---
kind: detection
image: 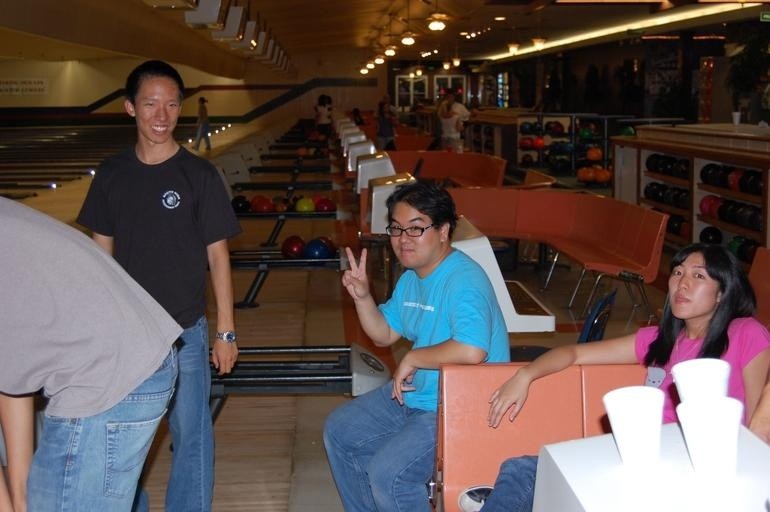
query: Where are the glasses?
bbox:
[386,221,438,237]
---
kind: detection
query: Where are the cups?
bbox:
[732,111,742,127]
[602,385,667,464]
[678,399,744,472]
[670,356,730,398]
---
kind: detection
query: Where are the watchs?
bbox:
[215,329,237,345]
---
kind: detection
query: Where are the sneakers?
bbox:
[457,486,495,512]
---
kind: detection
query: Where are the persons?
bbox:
[191,94,213,152]
[72,58,242,510]
[320,176,512,511]
[311,86,505,155]
[0,190,185,512]
[458,238,770,510]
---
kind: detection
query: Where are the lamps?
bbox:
[355,0,553,83]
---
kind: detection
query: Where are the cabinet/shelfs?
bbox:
[390,75,430,112]
[434,70,466,111]
[612,140,693,281]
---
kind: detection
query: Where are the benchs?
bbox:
[692,142,764,284]
